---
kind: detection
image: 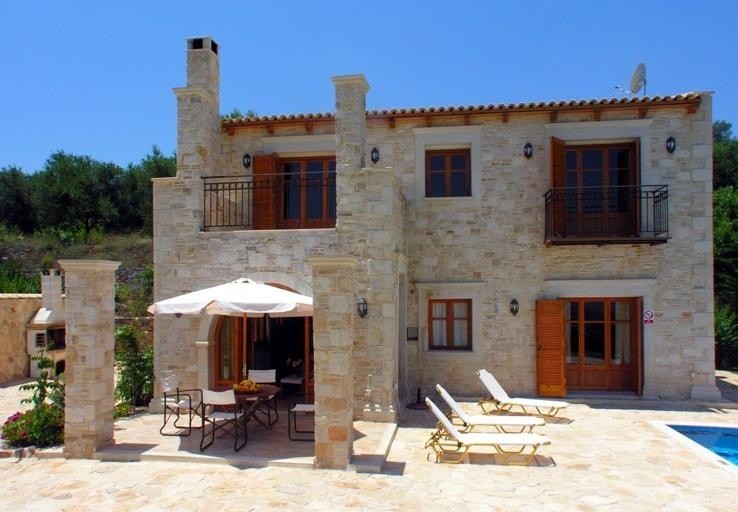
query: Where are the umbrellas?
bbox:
[142,273,313,386]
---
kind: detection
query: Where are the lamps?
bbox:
[357,297,368,318]
[509,299,520,316]
[666,136,675,154]
[243,152,250,168]
[371,146,379,164]
[524,142,533,160]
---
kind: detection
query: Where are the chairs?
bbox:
[159,369,314,452]
[424,367,571,467]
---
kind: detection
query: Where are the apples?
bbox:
[232,384,239,390]
